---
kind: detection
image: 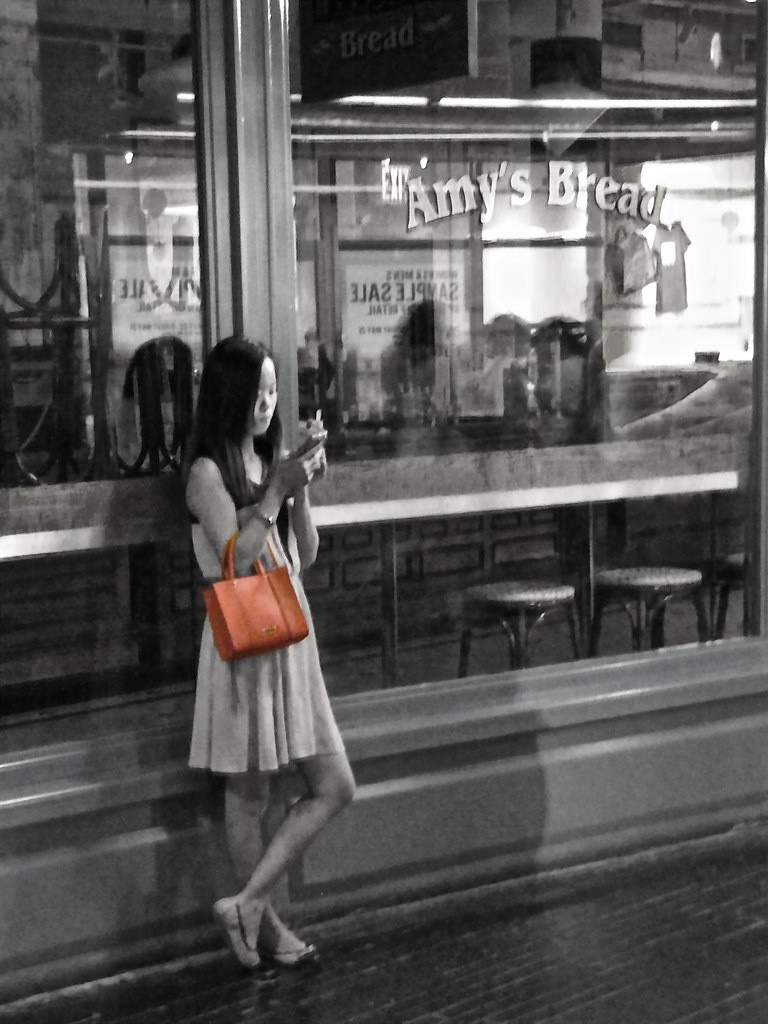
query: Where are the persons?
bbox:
[362,290,634,447]
[172,336,360,979]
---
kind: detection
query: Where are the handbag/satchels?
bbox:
[203,530,309,661]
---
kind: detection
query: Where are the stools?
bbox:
[457,580,584,678]
[714,551,753,640]
[585,565,711,658]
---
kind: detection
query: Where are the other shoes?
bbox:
[213,898,261,969]
[257,941,319,968]
